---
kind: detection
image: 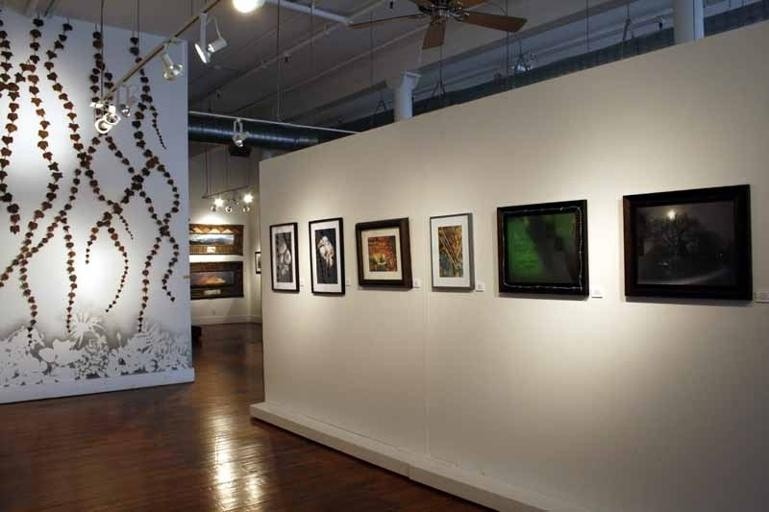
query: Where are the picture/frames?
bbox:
[623,185,752,300]
[355,218,412,288]
[430,213,475,290]
[255,251,261,274]
[270,222,299,292]
[497,200,588,295]
[309,218,345,294]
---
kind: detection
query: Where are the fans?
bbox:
[348,0,527,49]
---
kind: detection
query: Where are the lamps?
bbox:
[202,183,253,214]
[96,0,266,132]
[227,116,250,148]
